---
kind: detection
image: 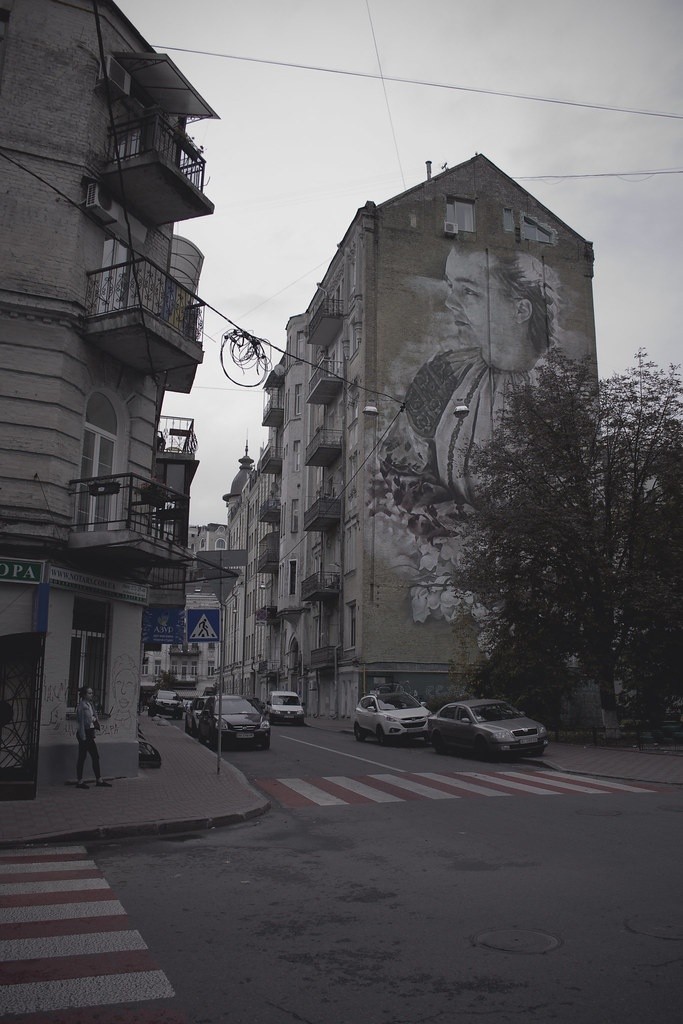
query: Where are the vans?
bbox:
[266,690,306,724]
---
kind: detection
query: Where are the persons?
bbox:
[255,701,270,720]
[381,227,599,624]
[76,685,112,789]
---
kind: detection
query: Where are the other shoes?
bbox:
[96,781,112,787]
[77,782,90,789]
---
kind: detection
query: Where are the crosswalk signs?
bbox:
[188,607,222,642]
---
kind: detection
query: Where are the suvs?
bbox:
[149,689,184,720]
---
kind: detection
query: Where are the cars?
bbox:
[197,695,271,749]
[185,700,192,711]
[184,695,208,737]
[353,691,434,746]
[428,698,548,759]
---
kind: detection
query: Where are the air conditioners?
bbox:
[443,222,460,235]
[85,182,120,223]
[98,53,131,97]
